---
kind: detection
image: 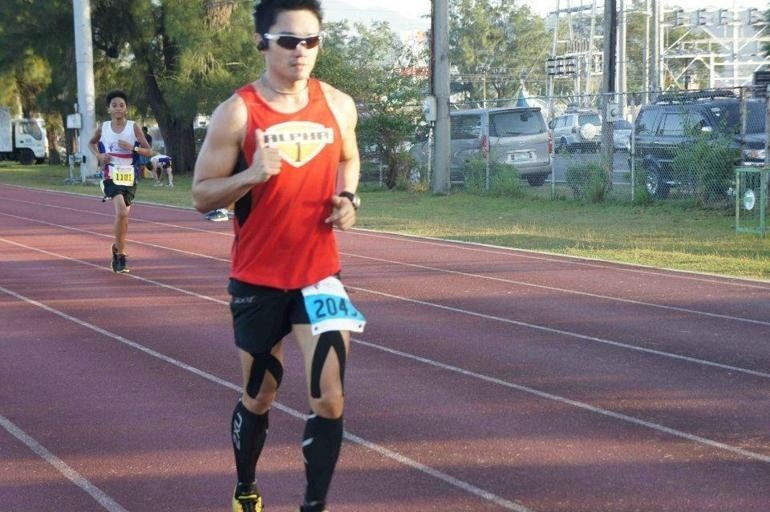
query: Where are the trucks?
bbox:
[0,110,49,166]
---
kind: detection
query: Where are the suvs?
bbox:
[627,86,770,203]
[546,108,609,156]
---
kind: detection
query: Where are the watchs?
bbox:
[338,192,361,210]
[134,146,138,153]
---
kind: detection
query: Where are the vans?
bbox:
[604,117,635,152]
[406,103,555,189]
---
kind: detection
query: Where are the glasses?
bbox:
[263,33,320,49]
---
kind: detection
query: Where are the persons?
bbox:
[88,89,152,274]
[139,127,152,165]
[147,154,175,188]
[191,0,367,512]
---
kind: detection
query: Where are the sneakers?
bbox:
[205,209,229,221]
[231,479,263,512]
[116,252,130,274]
[110,244,117,273]
